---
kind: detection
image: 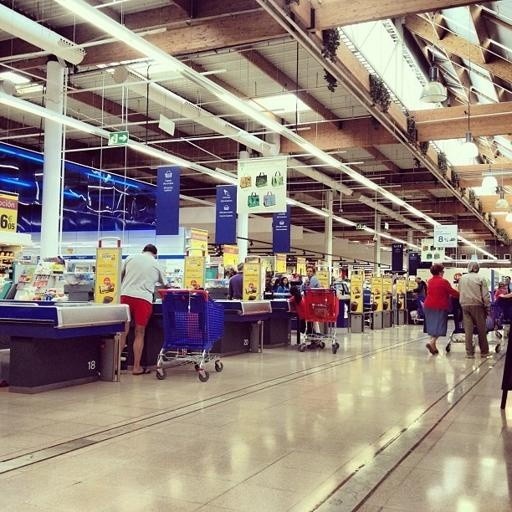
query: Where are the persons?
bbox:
[115,241,170,376]
[451,273,512,340]
[410,278,428,325]
[223,261,322,351]
[422,264,460,355]
[458,261,494,358]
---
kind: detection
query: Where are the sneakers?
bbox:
[426,343,438,355]
[308,343,320,349]
[465,353,491,358]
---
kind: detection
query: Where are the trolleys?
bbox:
[154,289,228,382]
[445,294,463,352]
[413,291,426,324]
[291,287,340,355]
[485,290,511,353]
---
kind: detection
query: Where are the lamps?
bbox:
[481,135,508,209]
[459,5,479,159]
[419,9,447,103]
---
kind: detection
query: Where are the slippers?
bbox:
[132,368,151,375]
